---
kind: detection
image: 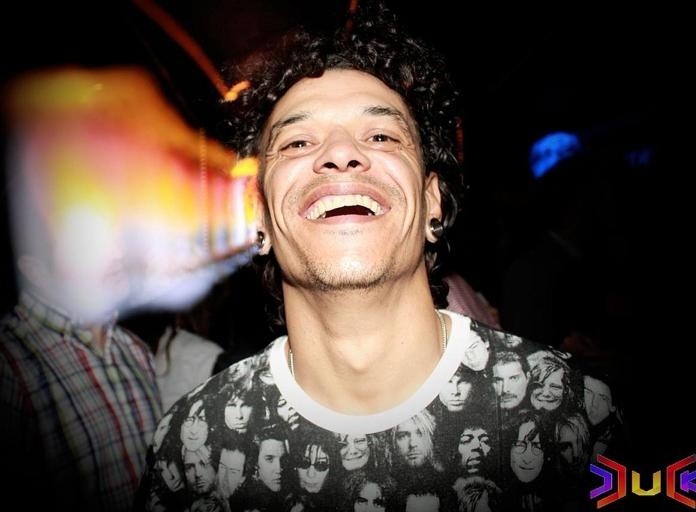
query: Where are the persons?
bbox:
[0,8,650,512]
